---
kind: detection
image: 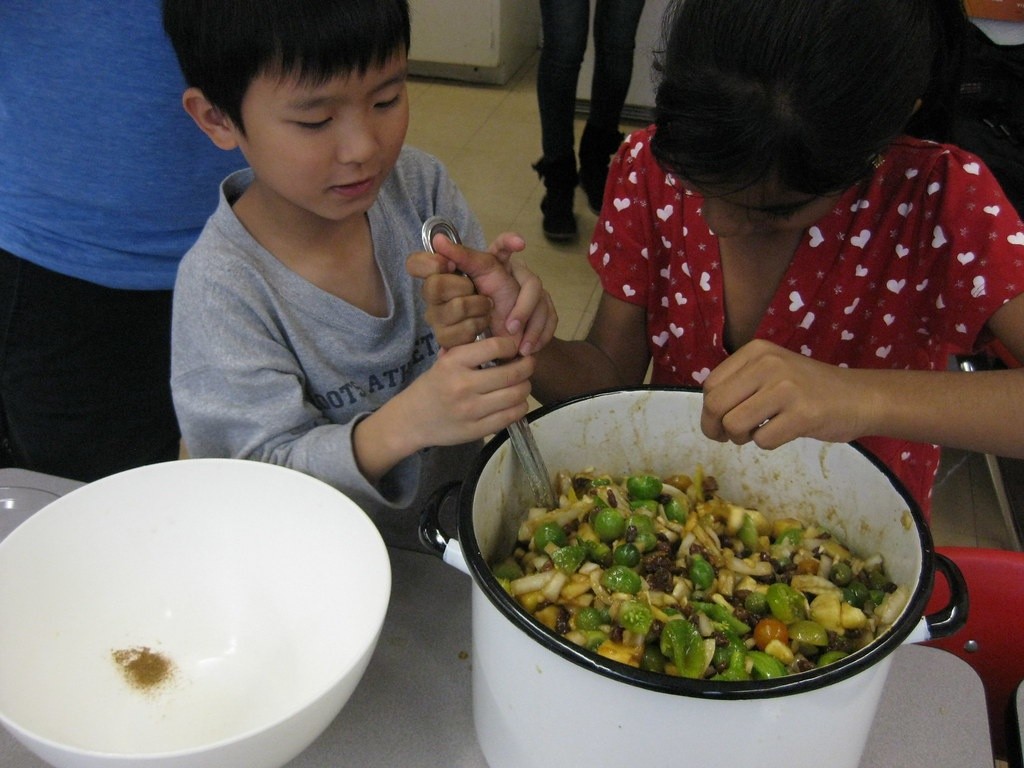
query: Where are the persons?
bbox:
[171,0,560,565]
[0,0,251,485]
[523,0,646,246]
[406,0,1023,531]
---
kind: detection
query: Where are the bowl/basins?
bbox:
[0,459,392,768]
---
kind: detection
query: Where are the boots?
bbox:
[581,133,620,213]
[541,171,576,243]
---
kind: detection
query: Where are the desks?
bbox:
[0,465,999,768]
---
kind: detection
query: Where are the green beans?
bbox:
[535,474,869,683]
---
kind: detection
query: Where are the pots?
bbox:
[419,384,970,768]
